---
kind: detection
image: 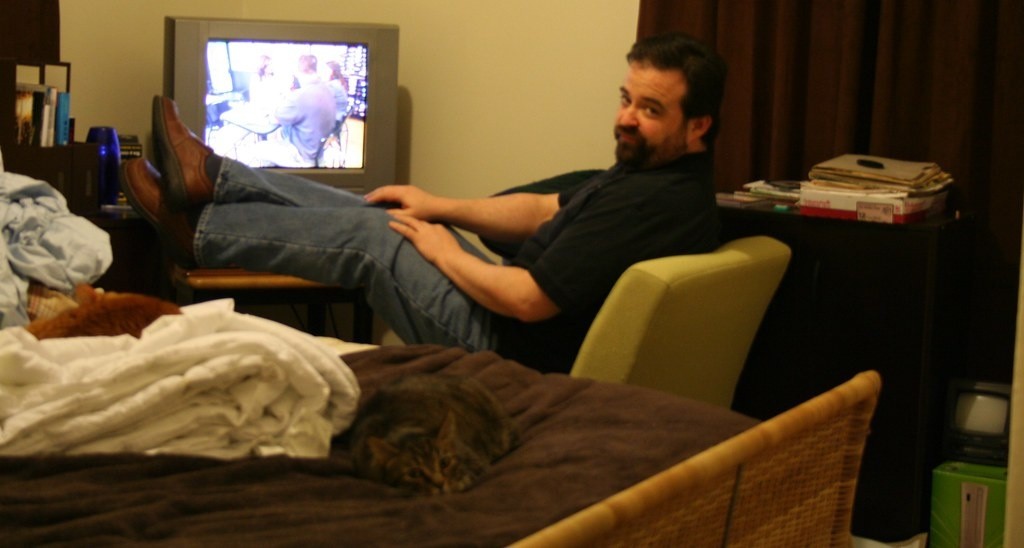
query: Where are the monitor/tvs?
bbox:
[162,16,399,193]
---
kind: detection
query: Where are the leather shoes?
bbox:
[118,157,194,254]
[153,94,214,212]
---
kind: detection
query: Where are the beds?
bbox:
[1,288,884,548]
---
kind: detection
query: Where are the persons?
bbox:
[259,53,337,165]
[326,59,348,130]
[120,30,726,376]
[259,53,275,80]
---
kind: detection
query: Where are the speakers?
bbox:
[85,124,120,206]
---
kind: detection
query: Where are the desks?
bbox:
[166,266,376,344]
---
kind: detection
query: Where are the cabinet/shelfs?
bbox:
[717,205,981,544]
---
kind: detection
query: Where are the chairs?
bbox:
[566,235,793,415]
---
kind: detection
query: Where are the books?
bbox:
[16,83,71,147]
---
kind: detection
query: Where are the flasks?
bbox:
[86,126,121,216]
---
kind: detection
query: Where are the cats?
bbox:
[326,368,524,495]
[25,284,183,341]
